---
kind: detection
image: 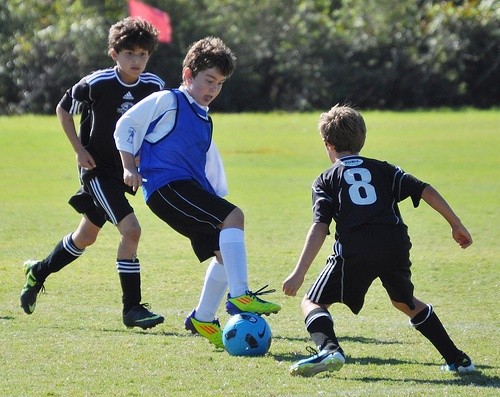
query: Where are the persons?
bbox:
[20,14,165,330]
[283,103,475,378]
[114,36,281,349]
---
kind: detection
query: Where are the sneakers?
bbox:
[441,351,474,373]
[20,260,45,314]
[123,303,164,330]
[184,309,226,349]
[225,285,282,316]
[289,345,345,377]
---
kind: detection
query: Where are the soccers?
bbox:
[222,313,272,357]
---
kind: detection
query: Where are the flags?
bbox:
[129,0,171,42]
